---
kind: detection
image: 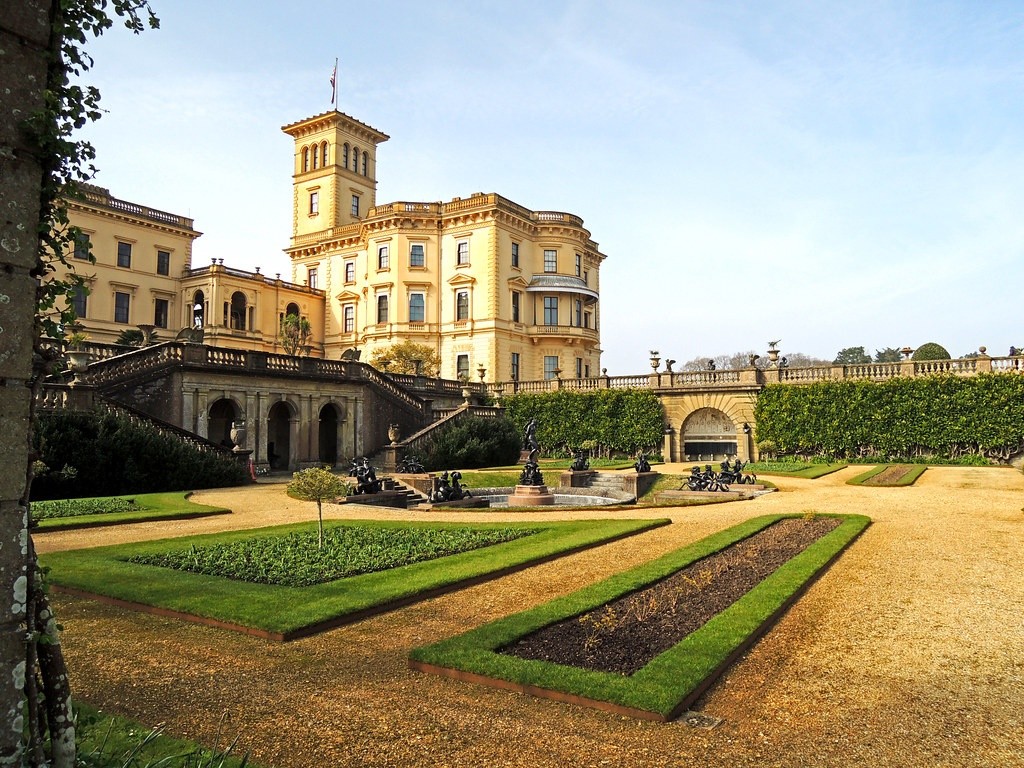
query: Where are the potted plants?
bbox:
[649,350,661,372]
[767,339,782,368]
[460,376,474,405]
[64,332,91,383]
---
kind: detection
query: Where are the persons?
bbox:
[524,420,539,462]
